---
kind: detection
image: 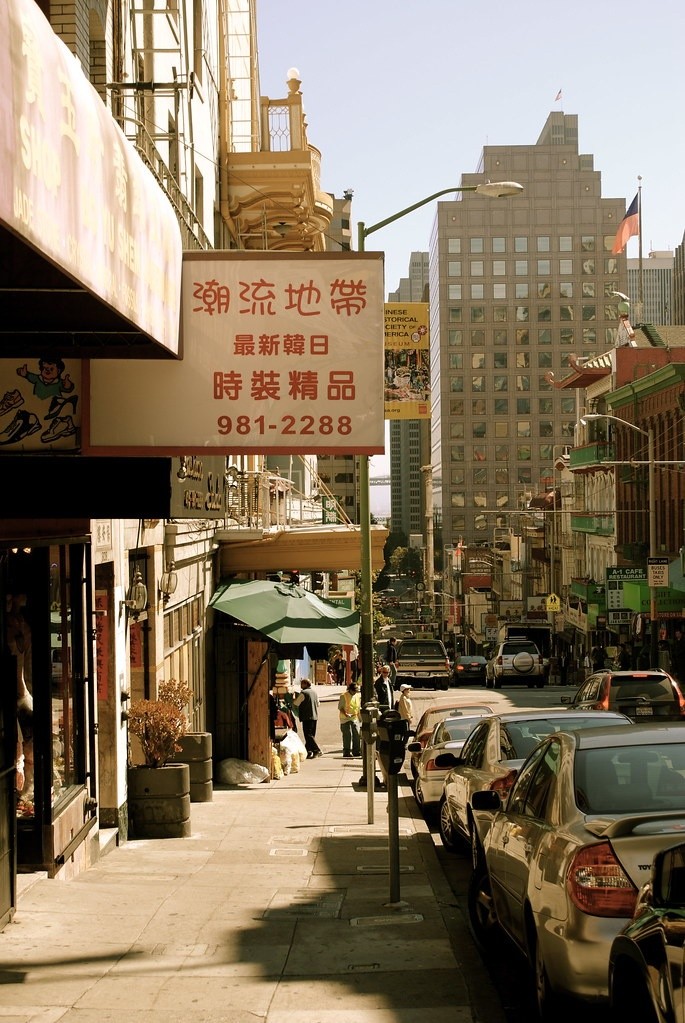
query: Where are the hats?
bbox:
[349,682,362,693]
[399,684,413,692]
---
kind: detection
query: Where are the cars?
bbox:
[408,703,497,780]
[403,631,413,639]
[434,709,674,896]
[465,720,685,1013]
[407,713,498,803]
[451,656,488,687]
[608,843,685,1023]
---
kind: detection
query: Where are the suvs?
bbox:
[561,668,685,723]
[485,636,544,688]
[394,639,451,691]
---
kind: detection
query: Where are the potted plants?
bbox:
[127,700,190,841]
[157,678,212,804]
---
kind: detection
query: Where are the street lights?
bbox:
[353,182,525,790]
[425,591,457,654]
[582,414,657,670]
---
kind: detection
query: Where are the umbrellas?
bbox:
[209,581,359,645]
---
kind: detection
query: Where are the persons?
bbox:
[338,682,361,757]
[568,655,578,687]
[583,651,597,680]
[618,644,631,671]
[333,654,345,685]
[293,677,323,759]
[375,665,394,713]
[670,628,685,685]
[558,651,568,685]
[398,684,413,750]
[386,636,398,686]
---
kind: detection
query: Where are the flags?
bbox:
[454,536,462,556]
[555,89,562,101]
[612,191,639,257]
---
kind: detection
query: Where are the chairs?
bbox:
[507,727,529,760]
[524,737,543,757]
[586,750,619,804]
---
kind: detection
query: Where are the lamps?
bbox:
[142,518,159,529]
[157,556,178,604]
[449,729,466,740]
[119,565,147,624]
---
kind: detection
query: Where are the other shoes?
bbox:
[343,753,353,757]
[307,751,323,759]
[353,752,362,756]
